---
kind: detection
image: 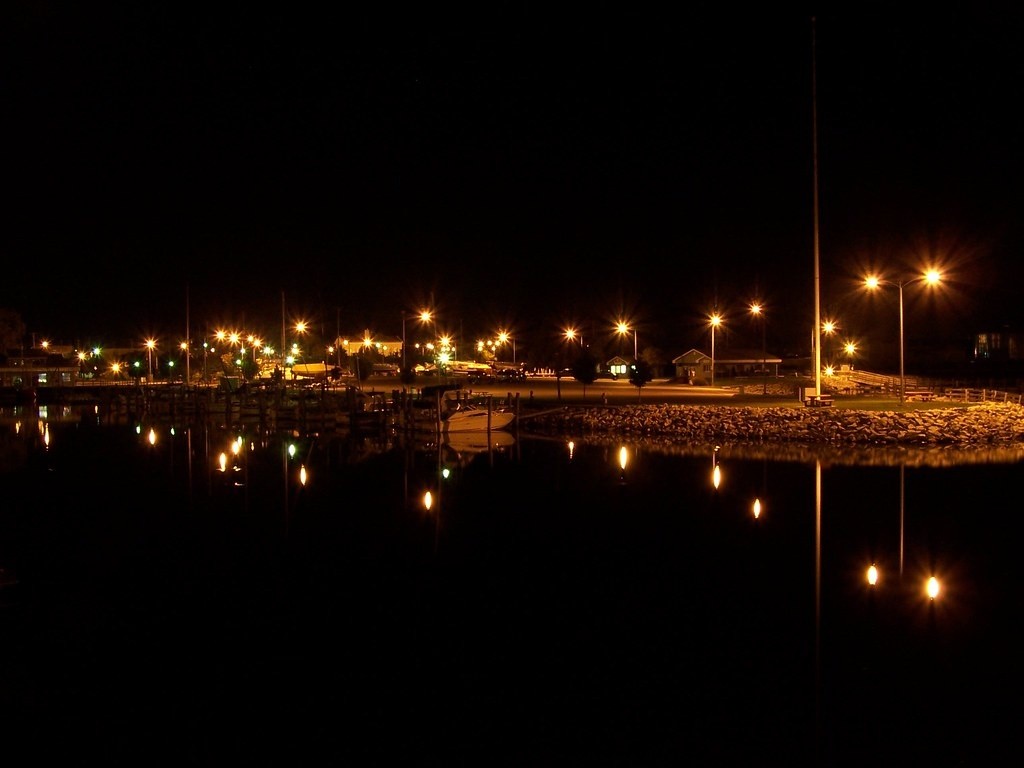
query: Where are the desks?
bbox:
[905,391,933,402]
[805,395,831,407]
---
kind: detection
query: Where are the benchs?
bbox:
[903,395,936,402]
[802,400,817,407]
[817,399,835,407]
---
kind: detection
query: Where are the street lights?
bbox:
[708,316,720,389]
[866,270,942,406]
[752,304,768,396]
[812,323,833,375]
[618,324,638,362]
[865,464,940,608]
[500,333,517,365]
[147,341,154,374]
